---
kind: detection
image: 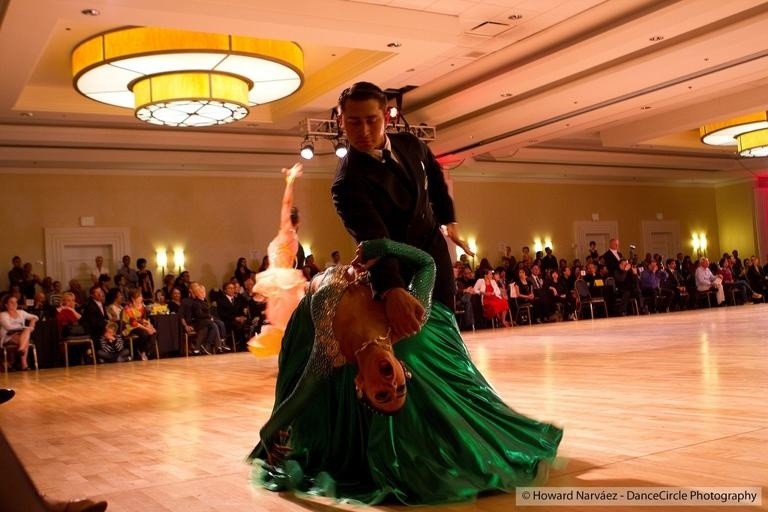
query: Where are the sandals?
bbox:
[41,492,107,512]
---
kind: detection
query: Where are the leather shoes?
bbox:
[190,337,231,355]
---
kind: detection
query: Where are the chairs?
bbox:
[0,270,768,374]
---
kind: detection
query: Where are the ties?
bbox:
[382,149,412,192]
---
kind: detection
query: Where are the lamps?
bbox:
[699,110,768,157]
[71,25,305,129]
[300,106,436,160]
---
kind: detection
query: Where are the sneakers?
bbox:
[137,350,149,361]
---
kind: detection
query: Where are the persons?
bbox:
[247,236,563,507]
[330,81,475,314]
[0,207,768,372]
